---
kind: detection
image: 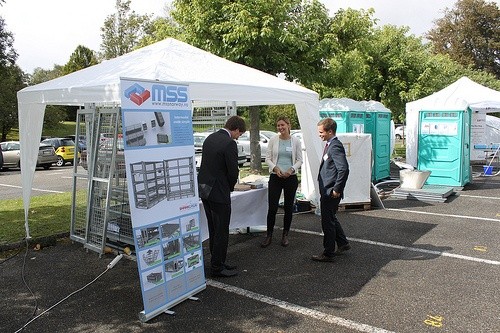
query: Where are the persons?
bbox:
[311,118,351,262]
[261,117,303,247]
[197,116,247,277]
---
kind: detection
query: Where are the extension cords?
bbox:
[106,253,124,269]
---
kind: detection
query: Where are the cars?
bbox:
[0,133,127,178]
[193,128,306,168]
[395,126,408,140]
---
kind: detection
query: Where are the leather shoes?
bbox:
[312,254,335,262]
[334,242,352,255]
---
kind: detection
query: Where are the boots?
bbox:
[261,230,274,248]
[282,229,289,246]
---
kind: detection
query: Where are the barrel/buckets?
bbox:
[483,166,493,175]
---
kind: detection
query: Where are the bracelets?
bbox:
[291,167,294,171]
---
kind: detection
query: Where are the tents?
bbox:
[406,76,500,168]
[18,37,322,239]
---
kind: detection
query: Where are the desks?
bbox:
[199,176,269,242]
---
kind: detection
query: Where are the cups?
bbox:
[124,247,131,255]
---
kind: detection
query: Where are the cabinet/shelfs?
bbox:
[130,156,195,210]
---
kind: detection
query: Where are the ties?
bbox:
[318,143,328,181]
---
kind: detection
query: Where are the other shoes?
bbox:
[211,267,237,276]
[223,264,237,270]
